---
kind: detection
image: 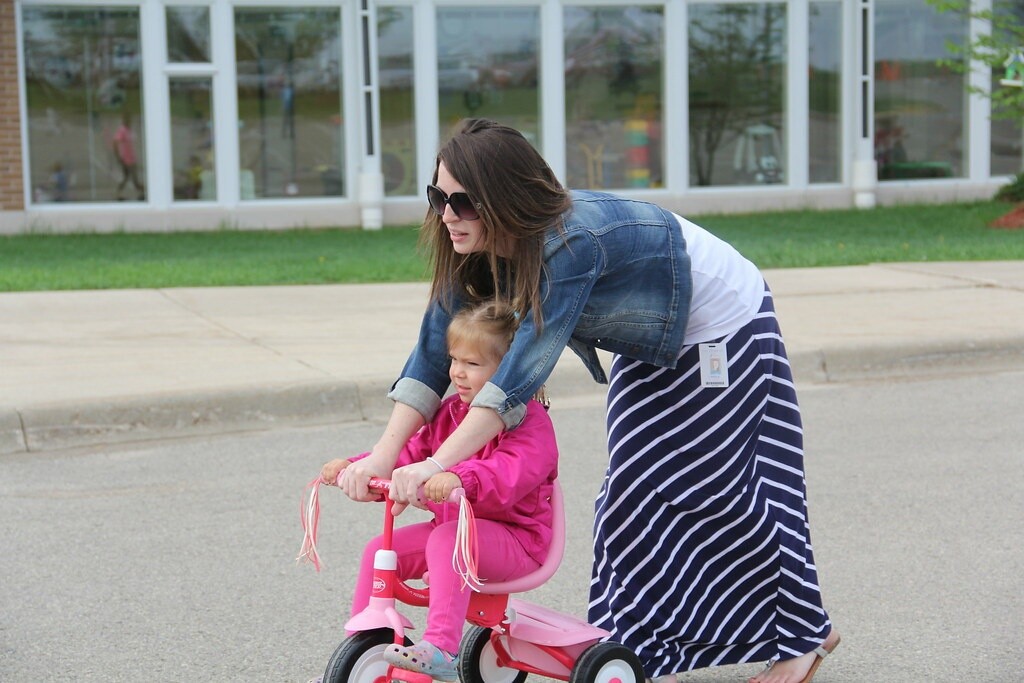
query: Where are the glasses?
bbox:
[427,184,480,221]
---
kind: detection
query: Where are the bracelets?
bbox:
[427,457,444,472]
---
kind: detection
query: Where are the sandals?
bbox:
[746,629,842,682]
[383,640,459,681]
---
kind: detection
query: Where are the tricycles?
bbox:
[321,468,644,683]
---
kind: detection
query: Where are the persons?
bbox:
[320,116,841,682]
[875,116,907,165]
[37,71,296,202]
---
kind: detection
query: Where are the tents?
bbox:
[488,17,662,87]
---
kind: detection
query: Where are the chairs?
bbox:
[423,479,566,595]
